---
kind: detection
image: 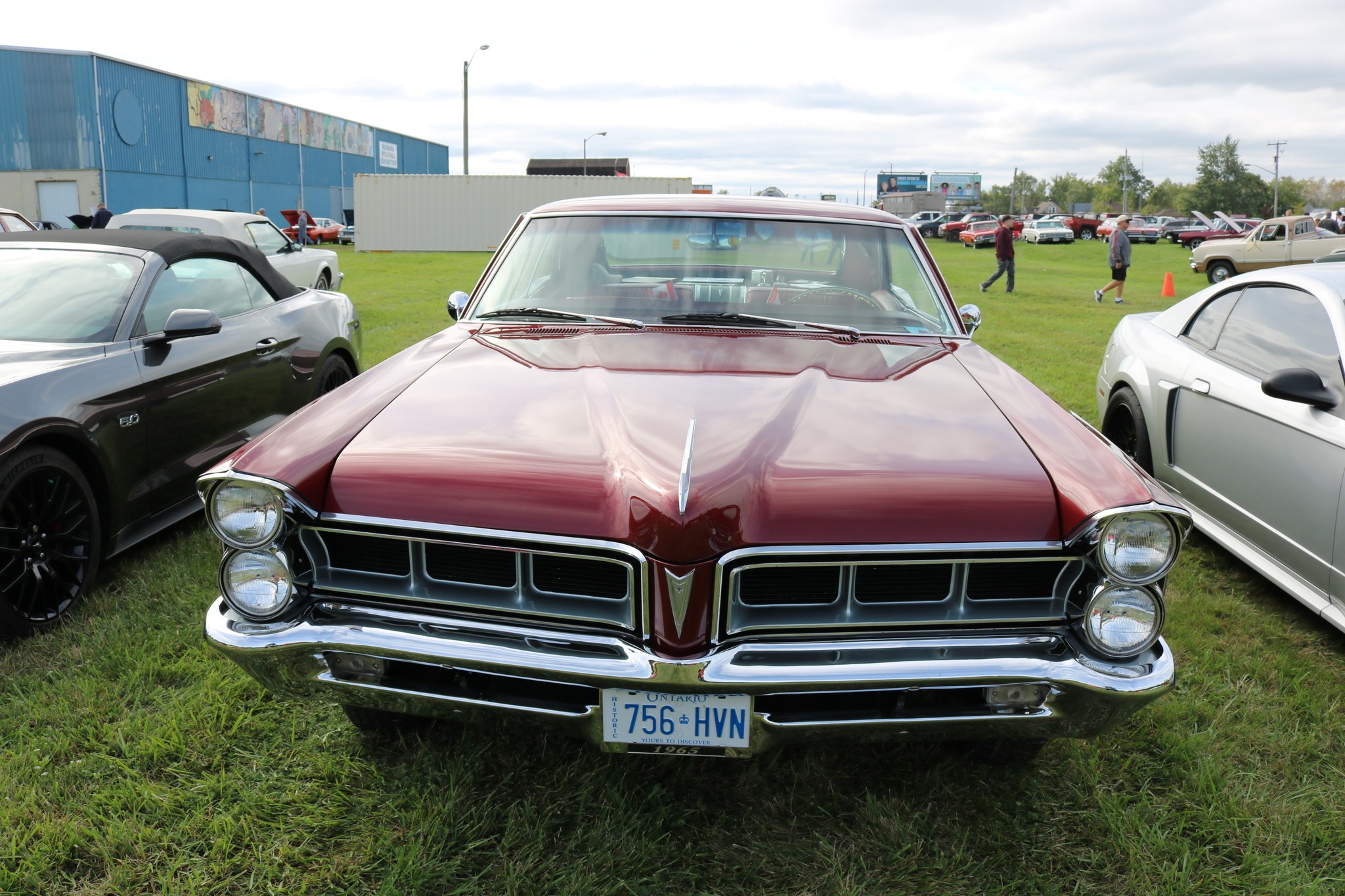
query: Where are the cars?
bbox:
[1096,212,1177,244]
[197,194,1192,763]
[938,213,998,242]
[0,205,363,633]
[337,226,355,245]
[1021,212,1121,245]
[278,209,346,245]
[1096,261,1345,635]
[1160,217,1276,250]
[959,214,1028,249]
[916,214,982,239]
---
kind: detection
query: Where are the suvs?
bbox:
[902,211,958,226]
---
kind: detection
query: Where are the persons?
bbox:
[799,246,815,266]
[90,202,113,229]
[1285,209,1292,216]
[256,208,266,216]
[1304,210,1345,234]
[979,215,1016,293]
[825,240,842,265]
[1093,214,1132,304]
[931,176,980,195]
[297,209,314,246]
[879,177,900,194]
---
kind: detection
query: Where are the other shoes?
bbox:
[311,242,314,245]
[300,243,303,245]
[980,283,986,292]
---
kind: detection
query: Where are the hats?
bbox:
[98,203,104,207]
[1001,215,1016,222]
[1117,215,1132,223]
[1336,212,1341,215]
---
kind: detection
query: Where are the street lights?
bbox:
[463,45,490,175]
[889,161,892,174]
[1241,163,1278,218]
[863,169,869,206]
[584,132,607,175]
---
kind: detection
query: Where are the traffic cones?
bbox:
[1161,272,1175,298]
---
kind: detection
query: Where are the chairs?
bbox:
[590,283,694,312]
[748,287,856,310]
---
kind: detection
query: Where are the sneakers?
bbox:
[1094,290,1102,303]
[1114,300,1123,304]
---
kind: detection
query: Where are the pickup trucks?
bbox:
[1189,214,1345,285]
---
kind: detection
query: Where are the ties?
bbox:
[1335,218,1338,221]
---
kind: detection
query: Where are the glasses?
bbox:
[1124,221,1129,223]
[1316,220,1320,221]
